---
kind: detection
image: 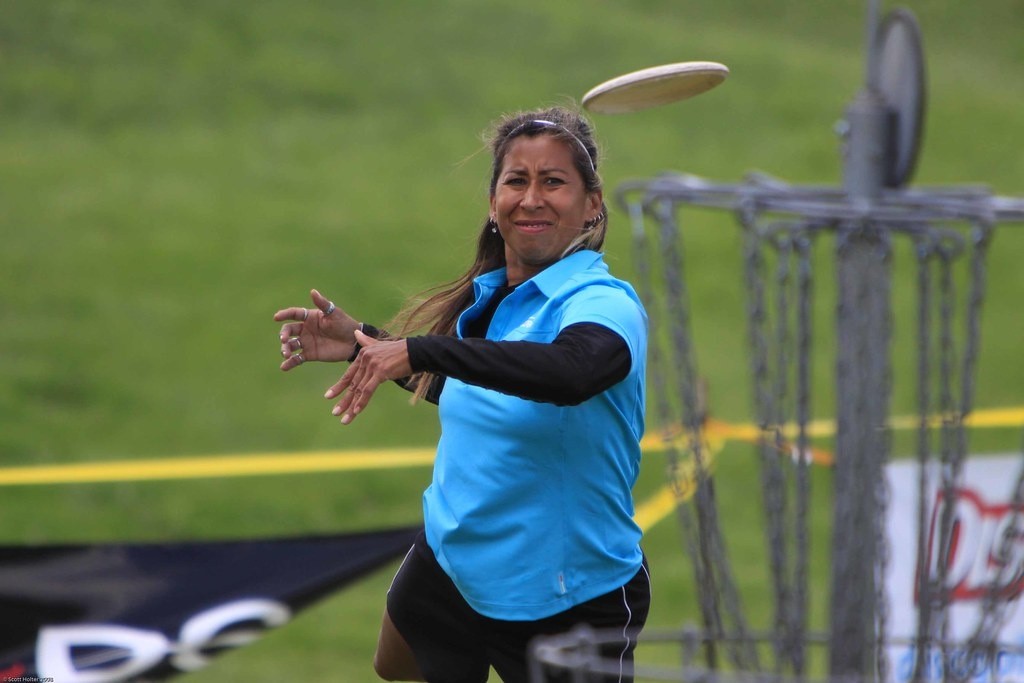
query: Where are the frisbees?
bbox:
[581,60,730,115]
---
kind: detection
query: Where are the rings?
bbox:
[324,302,335,314]
[292,338,301,348]
[296,354,303,363]
[303,308,307,320]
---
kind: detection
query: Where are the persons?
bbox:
[274,107,652,683]
